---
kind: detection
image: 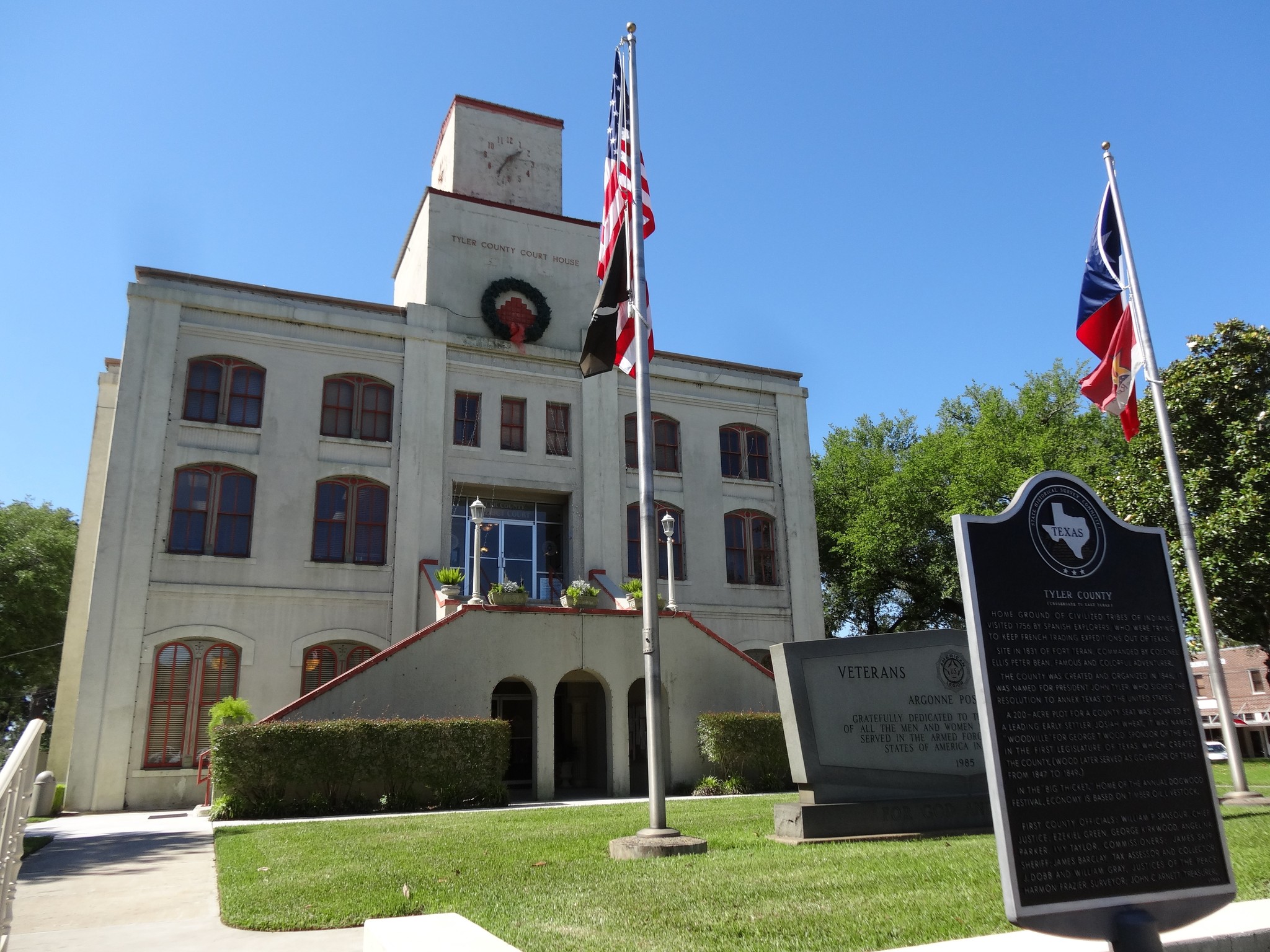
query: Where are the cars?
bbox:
[1205,740,1229,764]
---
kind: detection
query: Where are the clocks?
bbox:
[482,135,536,185]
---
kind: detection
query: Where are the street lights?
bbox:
[467,495,486,605]
[661,509,678,612]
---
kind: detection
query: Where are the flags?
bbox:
[577,42,658,382]
[1075,165,1148,442]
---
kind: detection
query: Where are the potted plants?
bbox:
[559,575,602,608]
[628,588,666,612]
[619,577,642,601]
[434,565,465,596]
[209,696,256,726]
[487,570,529,605]
